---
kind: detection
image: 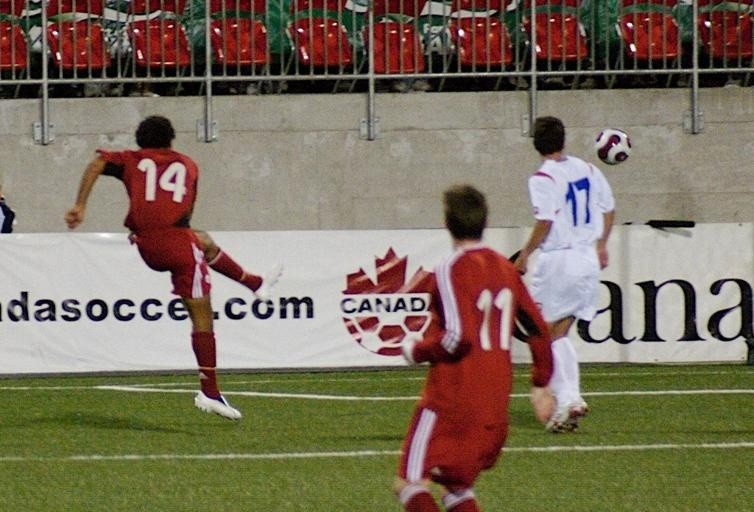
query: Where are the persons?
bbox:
[65,115,283,420]
[392,184,556,512]
[515,114,615,433]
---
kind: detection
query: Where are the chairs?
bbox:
[1,0,40,95]
[614,0,686,85]
[278,0,357,91]
[43,2,115,92]
[438,1,509,88]
[524,3,594,86]
[696,1,750,85]
[360,3,429,92]
[202,1,271,95]
[118,2,196,94]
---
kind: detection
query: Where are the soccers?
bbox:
[596,128,632,165]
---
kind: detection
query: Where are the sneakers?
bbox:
[547,397,590,432]
[194,389,244,418]
[253,260,283,303]
